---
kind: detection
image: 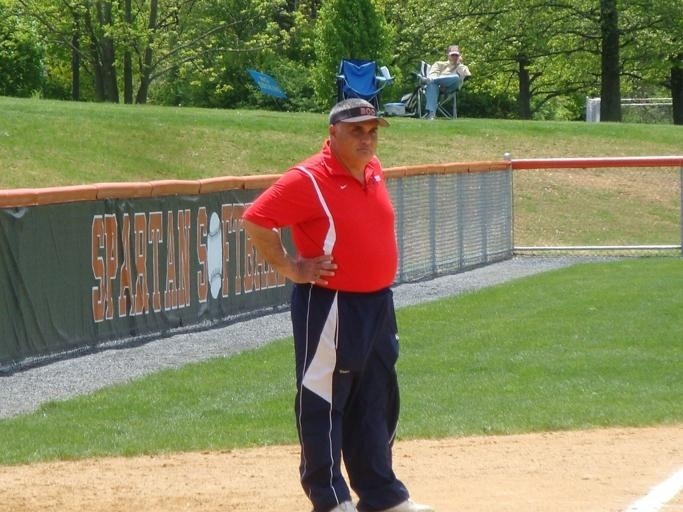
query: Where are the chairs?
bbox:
[337,55,395,117]
[411,59,473,121]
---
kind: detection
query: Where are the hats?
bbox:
[329,98,391,129]
[448,45,460,55]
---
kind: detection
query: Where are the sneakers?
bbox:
[421,112,436,120]
[380,498,435,512]
[330,499,359,512]
[417,74,429,89]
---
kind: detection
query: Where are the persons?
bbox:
[241,98,437,512]
[589,92,600,99]
[409,45,471,120]
